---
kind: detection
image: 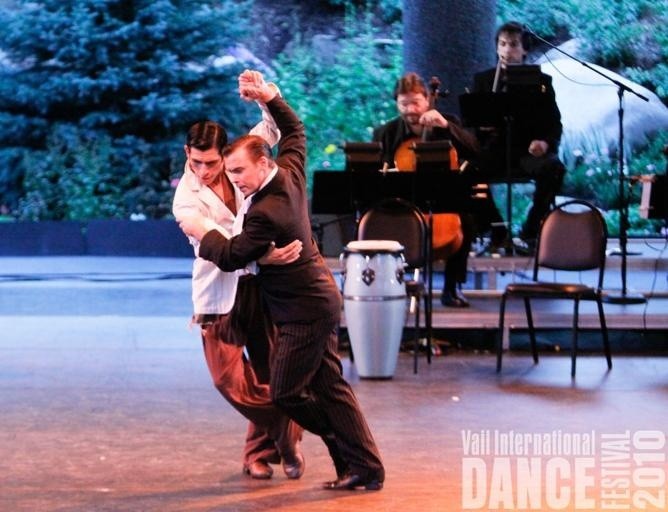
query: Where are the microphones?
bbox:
[521,29,538,52]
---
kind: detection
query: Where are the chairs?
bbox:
[338,197,615,377]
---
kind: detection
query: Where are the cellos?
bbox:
[395,76,464,263]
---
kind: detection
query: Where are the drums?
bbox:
[339,240,408,380]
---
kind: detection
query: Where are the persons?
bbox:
[171,67,306,480]
[474,21,566,258]
[376,72,481,310]
[174,82,385,493]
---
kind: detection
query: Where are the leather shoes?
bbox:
[283,430,305,478]
[243,459,274,478]
[322,469,384,490]
[442,287,471,306]
[476,231,509,257]
